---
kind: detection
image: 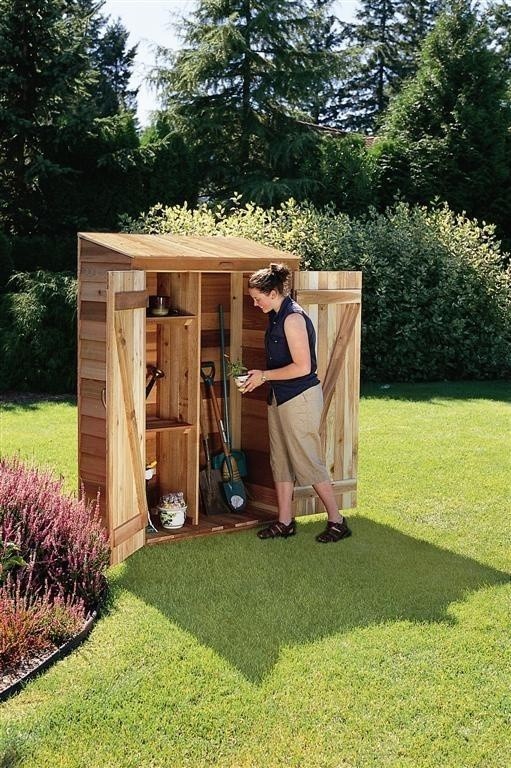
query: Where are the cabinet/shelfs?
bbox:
[144,314,195,433]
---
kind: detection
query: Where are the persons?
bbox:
[238,263,354,544]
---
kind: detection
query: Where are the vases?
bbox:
[155,503,188,530]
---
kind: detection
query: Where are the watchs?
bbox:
[261,372,268,383]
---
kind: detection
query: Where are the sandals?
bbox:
[257,521,295,539]
[317,517,351,543]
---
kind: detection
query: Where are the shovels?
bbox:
[199,361,252,516]
